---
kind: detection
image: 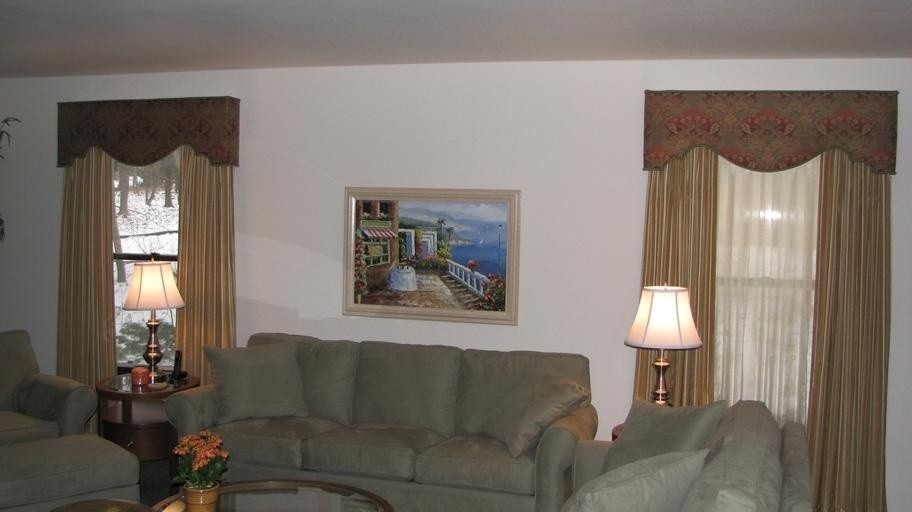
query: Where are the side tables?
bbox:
[96,370,200,461]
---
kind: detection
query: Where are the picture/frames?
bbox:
[344,186,521,326]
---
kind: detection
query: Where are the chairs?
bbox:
[50,498,153,512]
[0,330,93,439]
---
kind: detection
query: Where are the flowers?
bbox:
[172,429,229,489]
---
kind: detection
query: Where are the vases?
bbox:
[183,481,220,512]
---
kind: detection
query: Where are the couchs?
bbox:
[164,332,598,512]
[572,401,813,511]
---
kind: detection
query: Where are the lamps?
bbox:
[124,257,186,383]
[624,283,703,406]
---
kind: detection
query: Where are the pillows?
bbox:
[481,369,589,457]
[246,333,358,426]
[454,348,589,435]
[353,341,463,439]
[572,449,709,512]
[201,341,308,424]
[601,396,727,475]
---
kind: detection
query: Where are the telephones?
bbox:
[172,351,187,379]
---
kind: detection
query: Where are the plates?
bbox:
[147,382,168,389]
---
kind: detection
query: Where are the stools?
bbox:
[0,434,138,512]
